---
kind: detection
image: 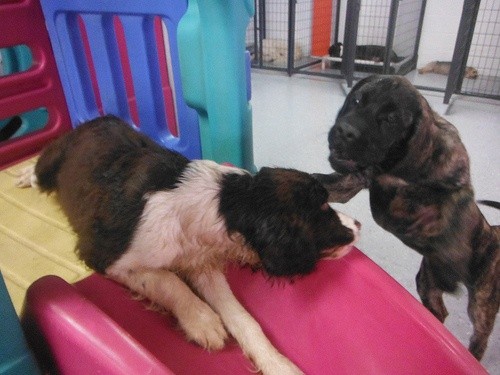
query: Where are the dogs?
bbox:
[250,39,304,66]
[418,60,477,79]
[329,42,407,63]
[310,74,500,362]
[17,114,362,375]
